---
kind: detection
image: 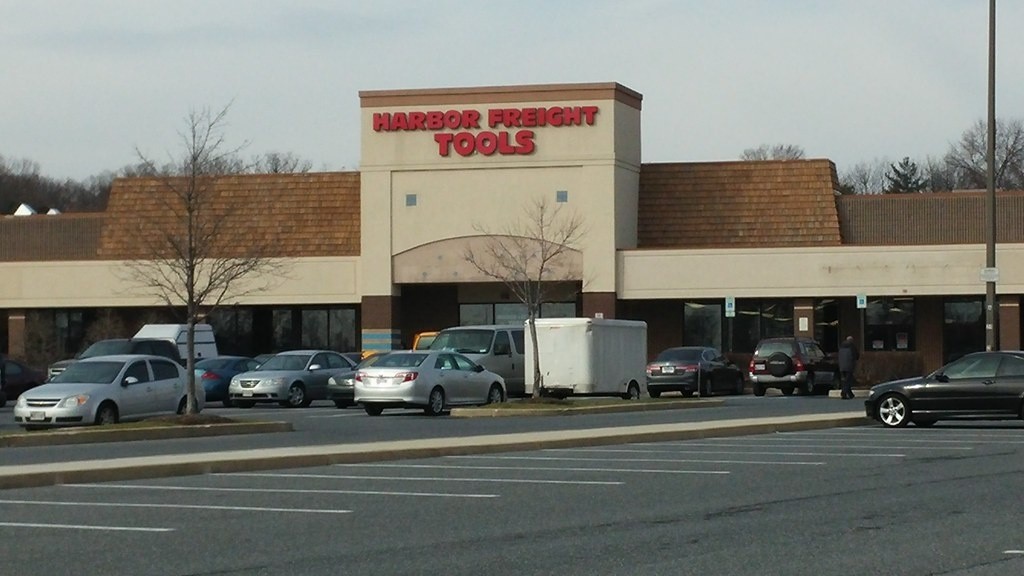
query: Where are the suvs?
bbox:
[46,336,181,383]
[748,336,836,397]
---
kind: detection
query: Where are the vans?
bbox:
[417,324,526,398]
[132,324,218,368]
[413,333,451,351]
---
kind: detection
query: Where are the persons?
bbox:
[838,336,860,400]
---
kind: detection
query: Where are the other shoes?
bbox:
[841,393,850,399]
[849,393,855,399]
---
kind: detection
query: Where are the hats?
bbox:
[846,336,853,341]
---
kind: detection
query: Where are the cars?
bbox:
[13,353,207,430]
[0,353,48,409]
[865,350,1024,428]
[185,354,262,405]
[228,350,359,409]
[354,348,508,417]
[645,346,744,398]
